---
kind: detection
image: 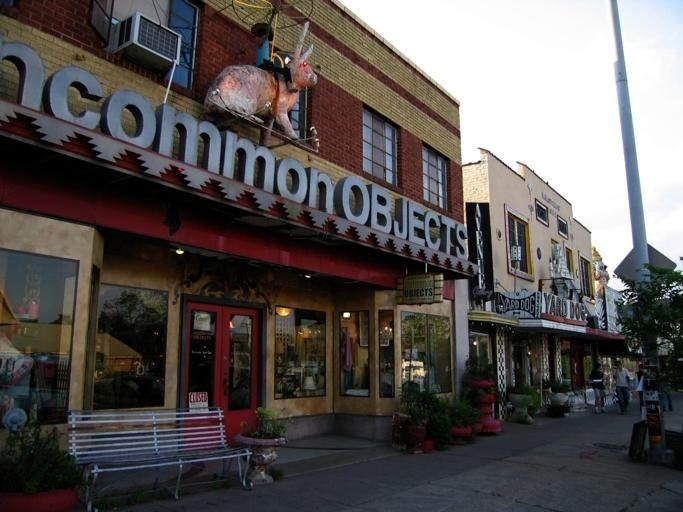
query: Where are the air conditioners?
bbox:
[109,11,182,74]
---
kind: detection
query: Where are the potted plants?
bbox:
[234,402,285,484]
[0,416,80,512]
[395,364,573,450]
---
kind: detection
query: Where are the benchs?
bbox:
[64,407,249,512]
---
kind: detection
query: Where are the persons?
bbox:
[637,365,644,416]
[613,361,630,415]
[590,364,608,414]
